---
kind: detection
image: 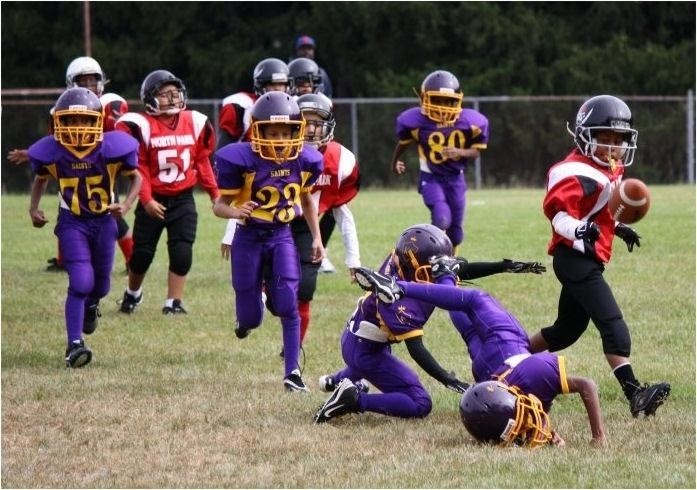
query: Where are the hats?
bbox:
[297,35,316,50]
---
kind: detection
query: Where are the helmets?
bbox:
[49,87,109,147]
[459,382,552,451]
[412,71,464,123]
[567,94,640,169]
[66,56,110,97]
[395,223,454,282]
[140,69,189,117]
[249,57,336,161]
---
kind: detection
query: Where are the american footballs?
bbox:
[609,178,650,224]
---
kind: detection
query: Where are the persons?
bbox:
[112,67,219,319]
[526,92,673,420]
[210,90,326,396]
[25,88,140,369]
[214,54,291,151]
[6,53,136,274]
[288,32,333,98]
[284,57,322,96]
[218,90,367,364]
[349,264,607,452]
[388,70,490,256]
[310,223,547,424]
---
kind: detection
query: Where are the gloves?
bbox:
[614,222,641,252]
[575,222,601,243]
[503,258,547,274]
[444,370,472,395]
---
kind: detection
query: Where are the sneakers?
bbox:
[283,373,311,393]
[116,287,143,316]
[65,339,93,369]
[235,291,268,339]
[628,380,671,417]
[318,257,336,273]
[427,254,469,278]
[318,375,369,394]
[162,298,187,315]
[314,377,361,425]
[354,266,405,305]
[83,300,102,334]
